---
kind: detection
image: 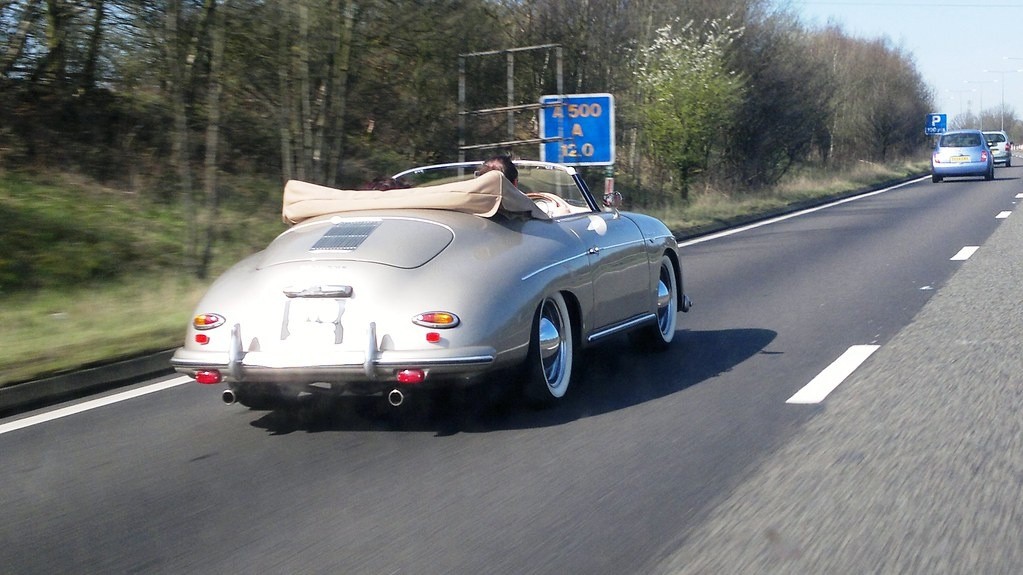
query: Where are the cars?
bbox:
[931,129,995,182]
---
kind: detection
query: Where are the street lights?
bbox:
[983,68,1023,131]
[946,89,976,129]
[963,80,999,130]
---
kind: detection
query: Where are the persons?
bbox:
[479,153,519,187]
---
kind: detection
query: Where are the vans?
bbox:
[971,131,1014,166]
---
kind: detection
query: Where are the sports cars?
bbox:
[168,159,695,411]
